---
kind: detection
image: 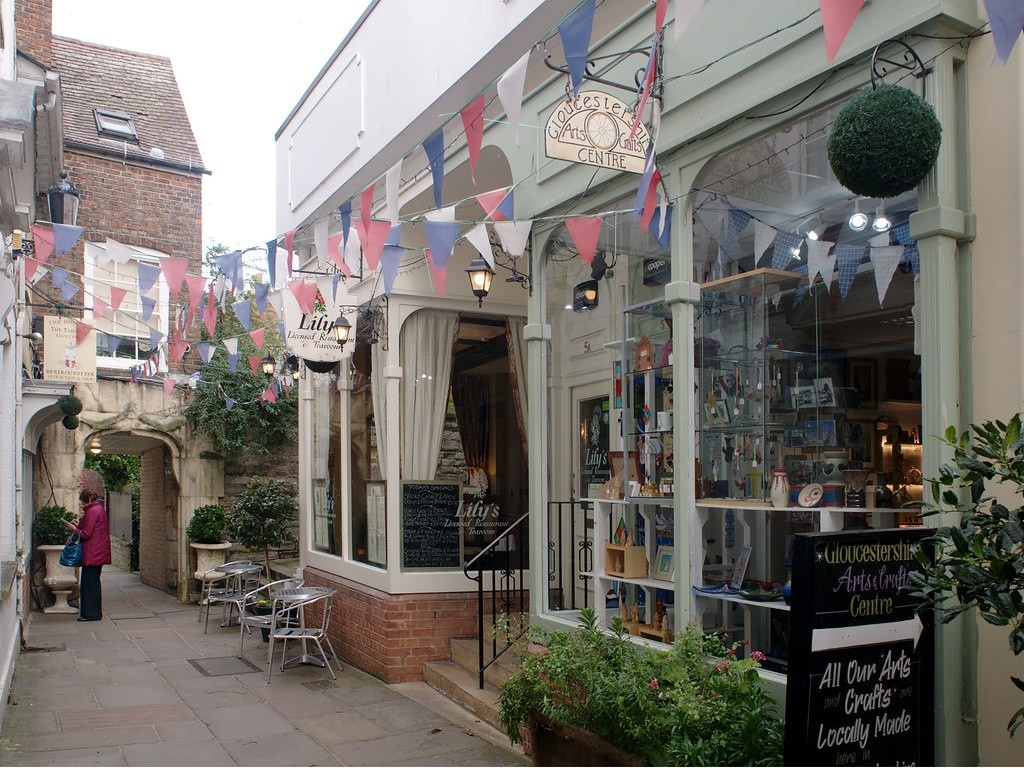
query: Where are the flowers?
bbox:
[488,606,785,767]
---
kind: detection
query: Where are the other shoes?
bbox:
[78,615,99,621]
[96,612,102,620]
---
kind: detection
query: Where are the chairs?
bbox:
[199,559,343,684]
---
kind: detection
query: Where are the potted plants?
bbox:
[188,475,298,642]
[34,505,79,614]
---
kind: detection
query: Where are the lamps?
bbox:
[260,346,306,380]
[790,209,829,260]
[136,138,164,159]
[464,230,532,308]
[333,295,389,351]
[12,169,80,260]
[849,193,892,232]
[89,447,102,453]
[28,343,41,365]
[16,331,43,345]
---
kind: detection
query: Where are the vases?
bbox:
[525,710,644,766]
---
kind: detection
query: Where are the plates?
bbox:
[798,482,823,508]
[690,583,784,602]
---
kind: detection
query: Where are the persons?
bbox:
[64,489,111,621]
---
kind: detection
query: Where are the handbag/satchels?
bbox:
[59,542,82,567]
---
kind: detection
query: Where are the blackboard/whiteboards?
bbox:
[782,526,940,767]
[399,479,465,572]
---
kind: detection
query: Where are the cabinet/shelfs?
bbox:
[579,270,925,676]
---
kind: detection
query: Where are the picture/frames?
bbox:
[652,546,675,581]
[703,399,731,423]
[846,358,879,411]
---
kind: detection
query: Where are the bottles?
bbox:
[770,469,792,508]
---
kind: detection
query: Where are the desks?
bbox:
[269,587,332,669]
[214,564,263,626]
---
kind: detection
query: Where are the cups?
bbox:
[656,411,673,431]
